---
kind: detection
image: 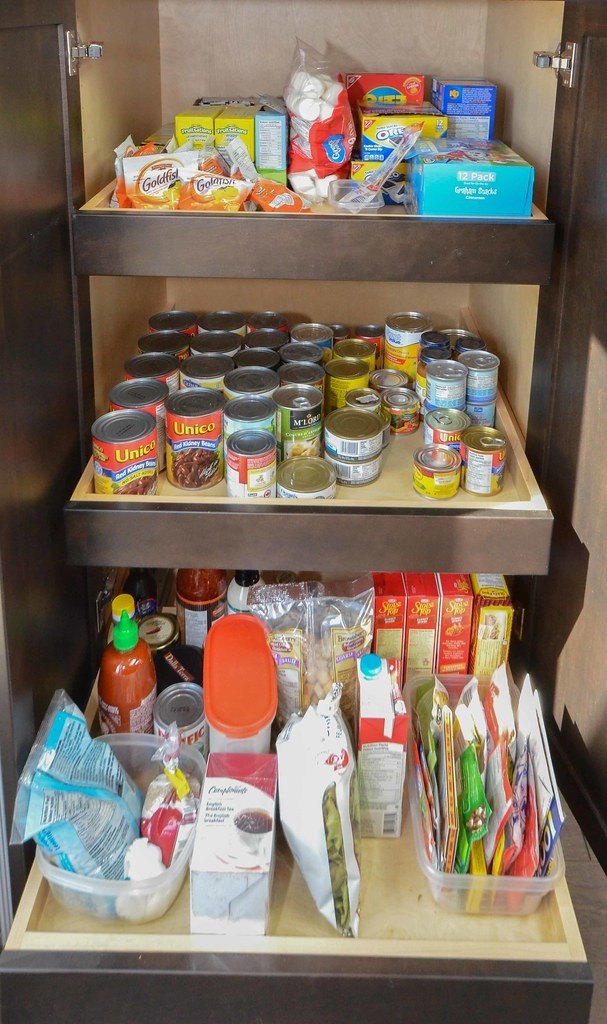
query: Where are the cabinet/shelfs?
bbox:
[0,157,594,1022]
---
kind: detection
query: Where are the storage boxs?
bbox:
[172,70,534,216]
[31,573,568,937]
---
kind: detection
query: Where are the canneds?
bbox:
[91,309,507,501]
[137,614,210,762]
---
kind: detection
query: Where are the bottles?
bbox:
[227,569,265,615]
[123,566,158,621]
[176,568,227,648]
[111,594,135,622]
[98,611,157,738]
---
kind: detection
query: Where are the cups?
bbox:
[233,809,272,851]
